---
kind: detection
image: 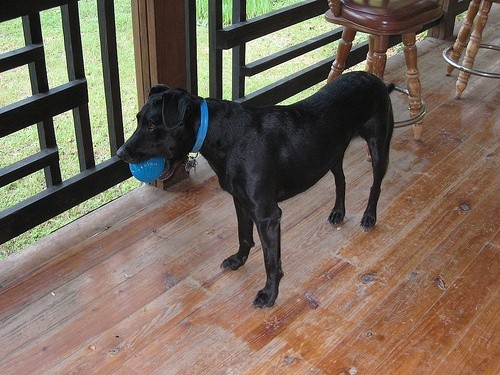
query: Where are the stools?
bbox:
[325,0,500,161]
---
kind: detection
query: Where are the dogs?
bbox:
[116,71,395,309]
